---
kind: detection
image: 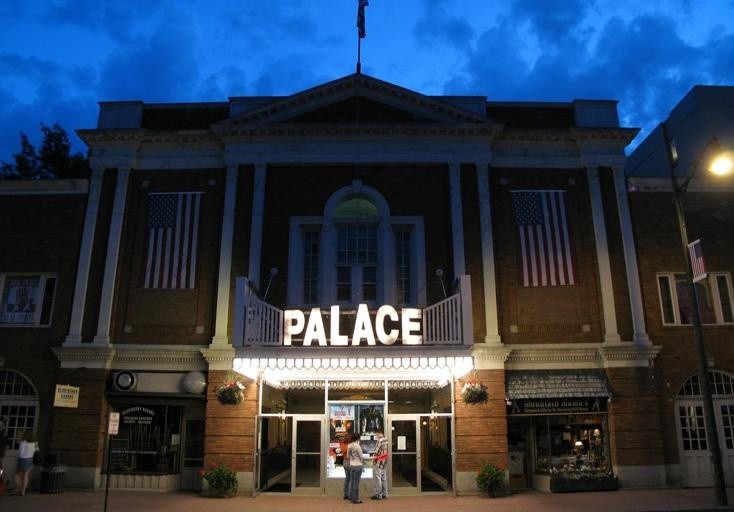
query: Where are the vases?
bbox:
[479,481,497,498]
[207,487,230,498]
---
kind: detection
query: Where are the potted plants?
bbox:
[473,456,507,490]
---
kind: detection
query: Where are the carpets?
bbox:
[263,482,302,492]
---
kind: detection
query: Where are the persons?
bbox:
[345,434,364,503]
[0,423,9,495]
[13,429,40,496]
[371,430,390,499]
[340,434,353,500]
[7,286,36,314]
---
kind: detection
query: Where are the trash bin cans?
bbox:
[39,464,67,494]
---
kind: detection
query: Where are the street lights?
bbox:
[659,120,733,504]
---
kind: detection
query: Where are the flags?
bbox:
[140,191,205,289]
[356,0,368,37]
[687,239,709,282]
[509,186,576,288]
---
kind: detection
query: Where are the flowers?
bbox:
[461,380,489,406]
[215,373,246,406]
[198,459,239,497]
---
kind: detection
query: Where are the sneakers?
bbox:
[371,494,386,500]
[344,496,363,504]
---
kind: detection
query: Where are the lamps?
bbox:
[593,428,602,447]
